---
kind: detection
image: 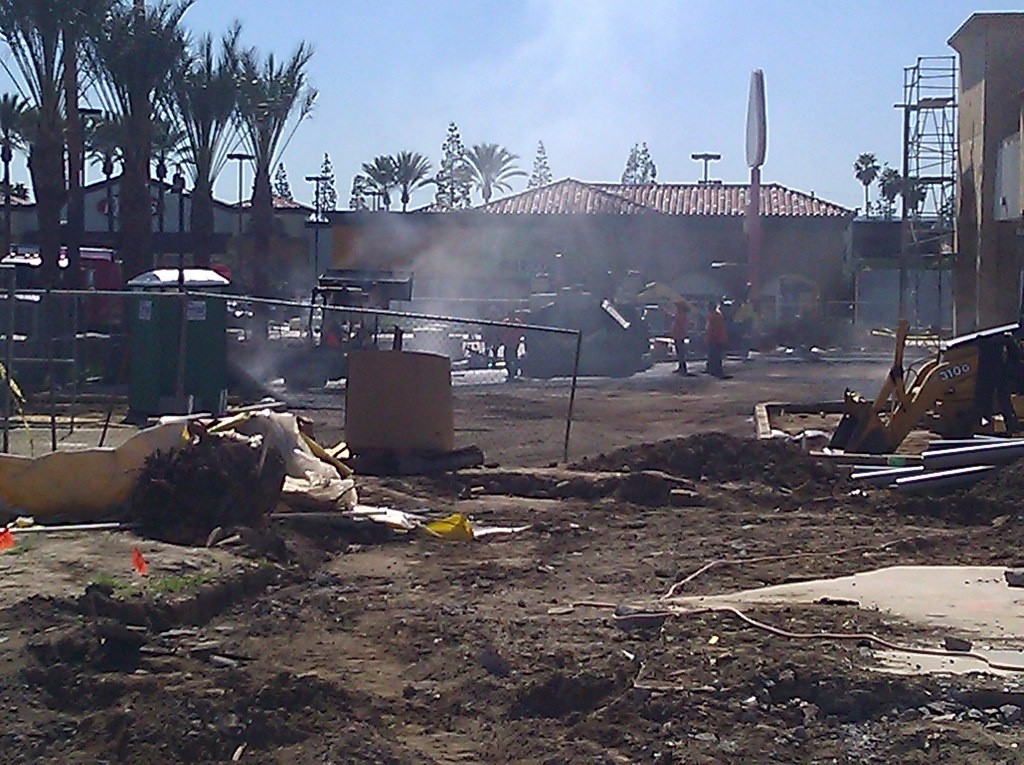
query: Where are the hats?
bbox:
[673,299,690,312]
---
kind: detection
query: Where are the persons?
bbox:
[661,299,695,376]
[482,303,523,379]
[700,301,726,378]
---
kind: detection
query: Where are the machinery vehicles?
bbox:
[790,316,1024,470]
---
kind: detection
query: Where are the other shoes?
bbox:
[702,370,710,373]
[672,369,680,373]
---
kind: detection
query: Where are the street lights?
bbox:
[76,108,102,249]
[226,153,257,295]
[306,174,333,300]
